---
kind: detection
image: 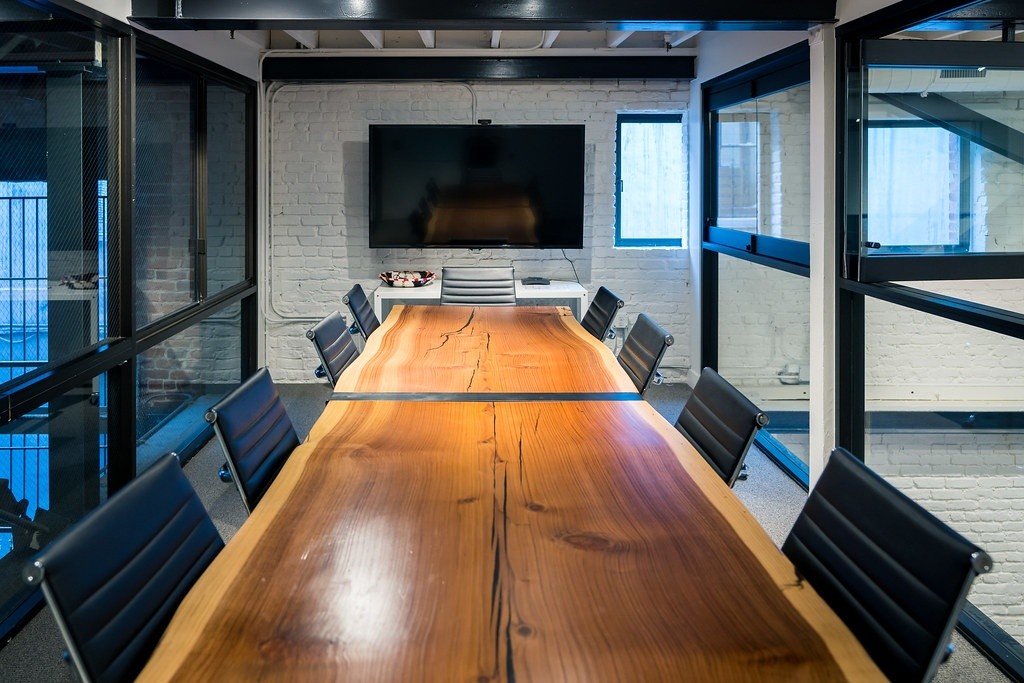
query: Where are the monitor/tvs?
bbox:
[367,123,584,249]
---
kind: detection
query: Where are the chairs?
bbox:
[20,452,226,683]
[675,366,770,490]
[205,367,301,514]
[306,310,360,389]
[782,447,992,683]
[440,266,517,306]
[343,284,380,341]
[580,286,625,342]
[616,311,675,397]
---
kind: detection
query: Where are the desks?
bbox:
[138,304,893,683]
[374,280,588,325]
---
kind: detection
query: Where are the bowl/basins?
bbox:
[379,271,435,288]
